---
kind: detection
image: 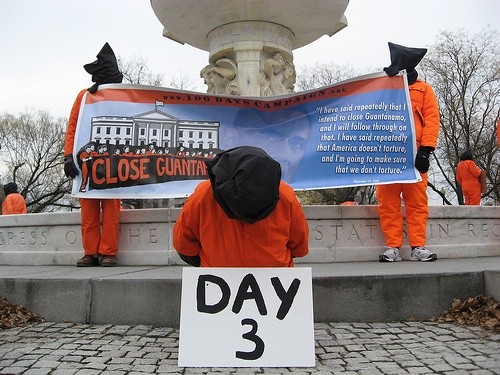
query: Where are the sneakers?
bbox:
[379,247,402,262]
[409,245,437,262]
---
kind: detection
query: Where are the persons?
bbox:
[339,197,357,206]
[63,42,123,268]
[2,182,28,215]
[456,150,481,205]
[375,41,440,261]
[225,103,312,183]
[172,146,309,267]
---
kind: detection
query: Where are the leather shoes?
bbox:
[100,255,117,266]
[77,254,100,267]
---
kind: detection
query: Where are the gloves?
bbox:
[415,146,432,173]
[64,154,79,178]
